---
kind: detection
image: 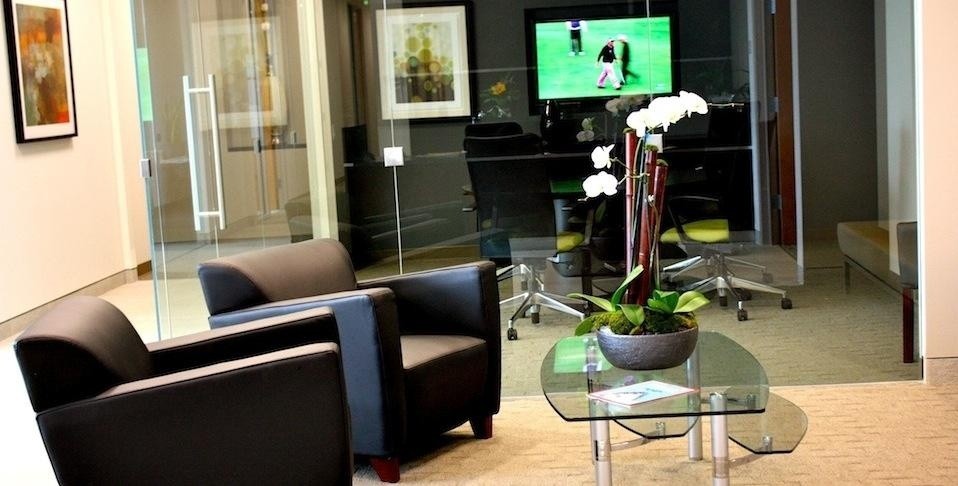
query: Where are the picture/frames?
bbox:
[4,0,79,148]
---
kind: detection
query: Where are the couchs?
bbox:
[14,291,352,486]
[198,240,501,483]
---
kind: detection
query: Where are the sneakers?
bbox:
[596,83,623,92]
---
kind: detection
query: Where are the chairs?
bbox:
[464,99,795,342]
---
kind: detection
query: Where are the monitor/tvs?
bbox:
[523,0,682,116]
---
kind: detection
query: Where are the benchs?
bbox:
[836,213,920,366]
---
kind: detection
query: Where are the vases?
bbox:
[595,315,698,370]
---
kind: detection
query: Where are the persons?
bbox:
[617,33,640,84]
[565,20,587,57]
[594,37,622,90]
